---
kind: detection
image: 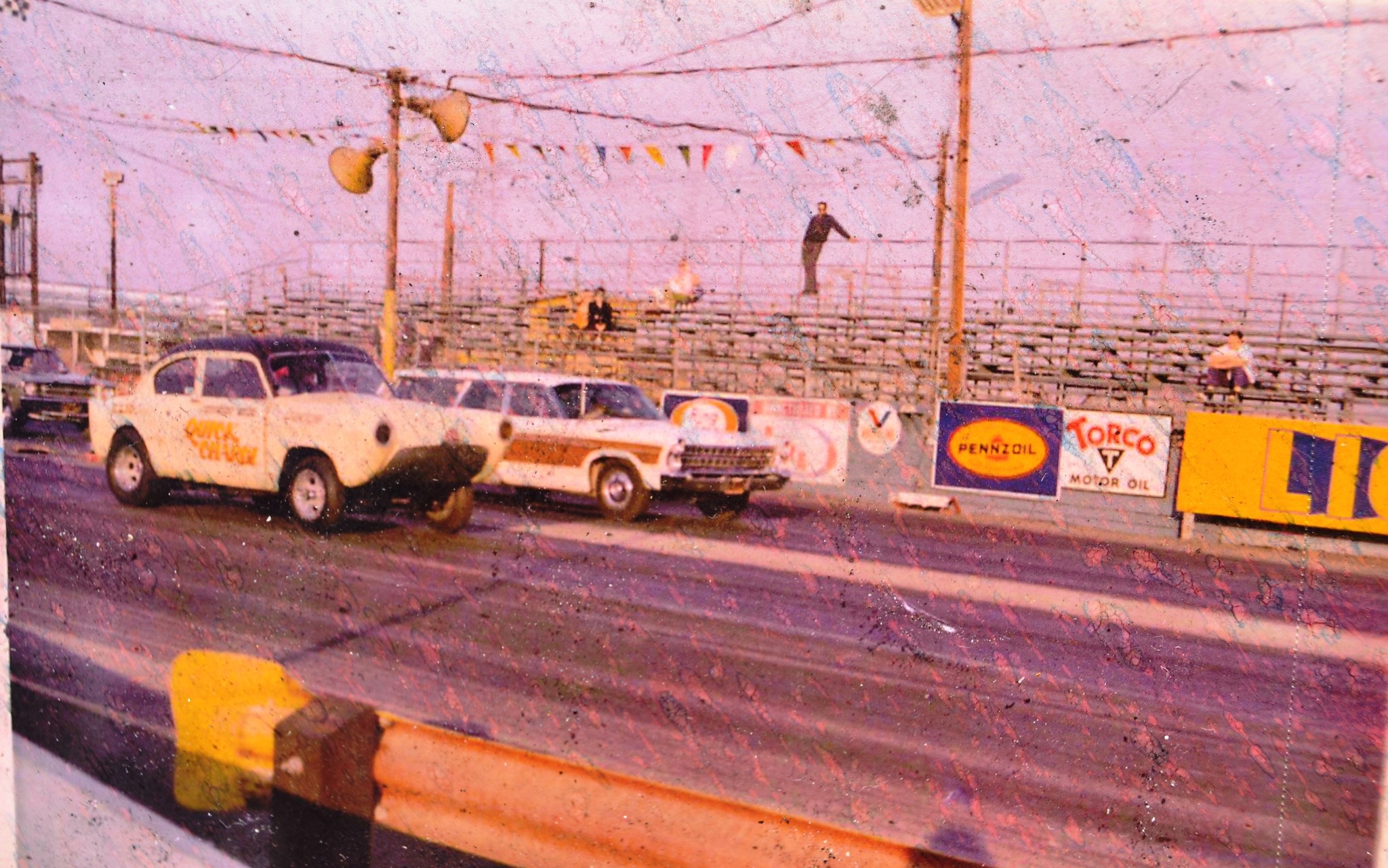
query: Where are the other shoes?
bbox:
[800,289,818,295]
[1229,391,1244,404]
[1198,390,1213,401]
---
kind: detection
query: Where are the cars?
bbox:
[376,367,788,523]
[2,344,115,438]
[87,338,490,532]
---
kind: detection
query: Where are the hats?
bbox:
[1222,330,1243,339]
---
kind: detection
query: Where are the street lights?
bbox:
[102,168,124,324]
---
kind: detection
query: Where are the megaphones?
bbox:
[330,143,387,193]
[406,88,469,142]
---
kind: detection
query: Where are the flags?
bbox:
[482,137,848,170]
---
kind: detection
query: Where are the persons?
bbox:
[1198,330,1255,403]
[582,287,616,331]
[798,201,855,296]
